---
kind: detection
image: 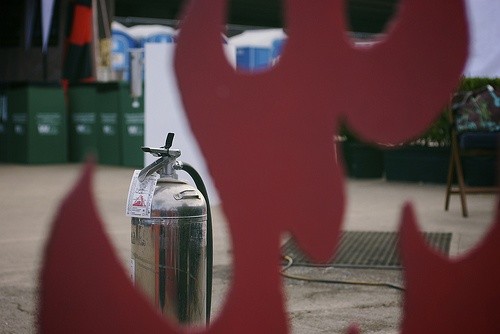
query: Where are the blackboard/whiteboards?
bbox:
[449,89,499,192]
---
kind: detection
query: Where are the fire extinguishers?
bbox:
[124,133,214,331]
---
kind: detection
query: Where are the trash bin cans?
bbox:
[69,80,104,164]
[97,82,127,168]
[121,85,145,170]
[24,82,70,166]
